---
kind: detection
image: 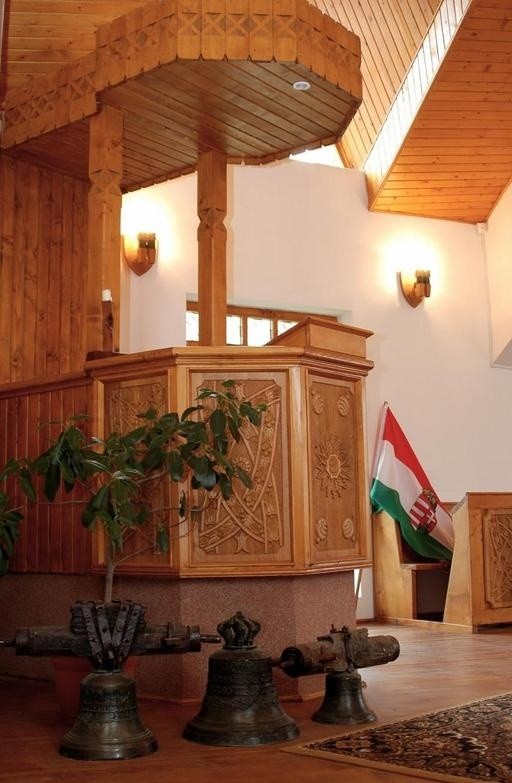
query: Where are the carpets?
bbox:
[279,691,511,782]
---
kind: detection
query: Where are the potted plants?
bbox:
[0,376,271,762]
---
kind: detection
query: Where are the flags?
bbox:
[370,406,454,560]
